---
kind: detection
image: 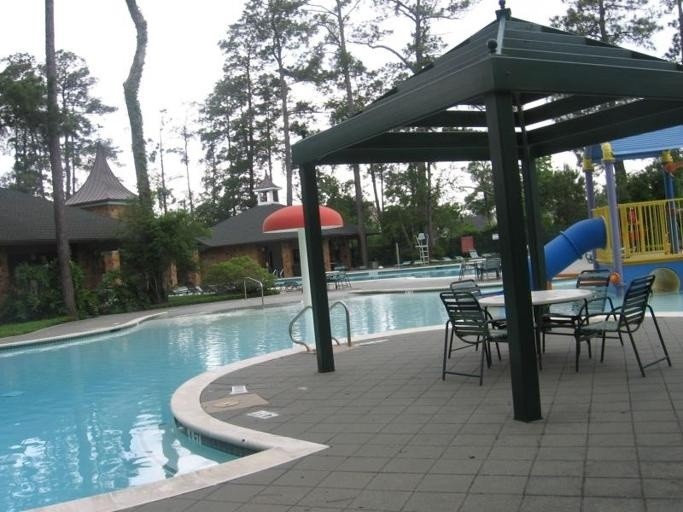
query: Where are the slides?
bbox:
[528,217,606,291]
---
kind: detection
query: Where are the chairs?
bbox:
[457,255,502,281]
[438,269,667,388]
[282,263,353,294]
